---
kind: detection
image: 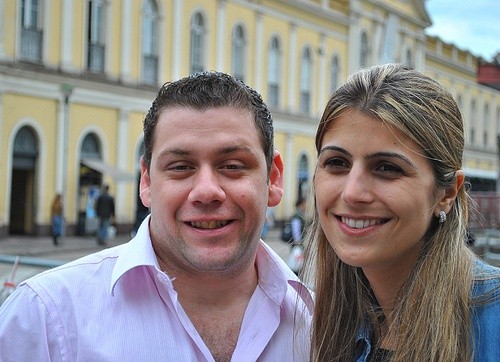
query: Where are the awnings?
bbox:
[82,159,131,184]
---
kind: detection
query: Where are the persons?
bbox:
[0,70,314,362]
[293,63,500,362]
[290,198,307,276]
[94,186,116,245]
[260,207,277,242]
[51,194,66,247]
